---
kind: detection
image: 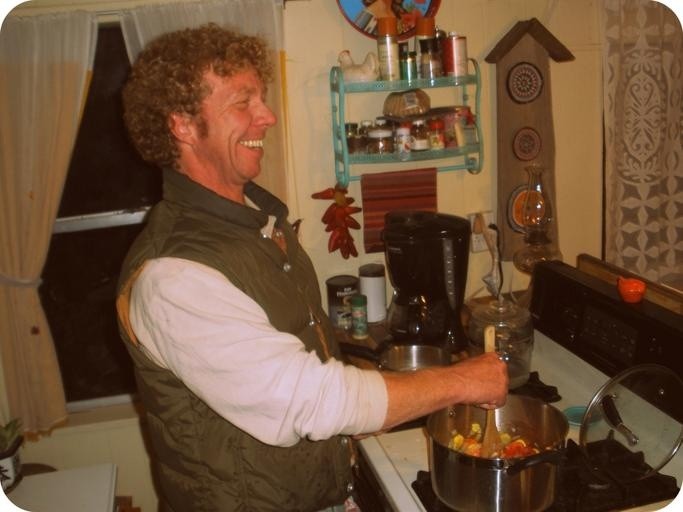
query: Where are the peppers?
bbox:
[311,186,361,259]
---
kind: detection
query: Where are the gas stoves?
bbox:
[411,436,680,512]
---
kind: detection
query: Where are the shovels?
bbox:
[478,325,506,458]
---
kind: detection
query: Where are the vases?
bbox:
[511,163,563,313]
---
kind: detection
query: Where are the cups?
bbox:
[617,276,647,303]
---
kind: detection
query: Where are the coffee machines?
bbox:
[379,211,472,355]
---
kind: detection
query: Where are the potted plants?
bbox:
[0,416,25,495]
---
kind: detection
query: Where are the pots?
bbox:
[335,338,454,375]
[425,393,571,512]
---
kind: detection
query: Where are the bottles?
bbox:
[356,262,387,324]
[345,115,445,164]
[399,51,417,81]
[349,294,370,341]
[411,15,469,80]
[468,293,535,391]
[376,15,400,83]
[326,271,361,335]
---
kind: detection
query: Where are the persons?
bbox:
[111,22,511,512]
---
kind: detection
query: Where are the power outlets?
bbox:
[467,210,498,254]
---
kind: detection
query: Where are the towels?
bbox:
[359,169,437,256]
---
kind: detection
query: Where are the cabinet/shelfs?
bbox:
[329,57,484,189]
